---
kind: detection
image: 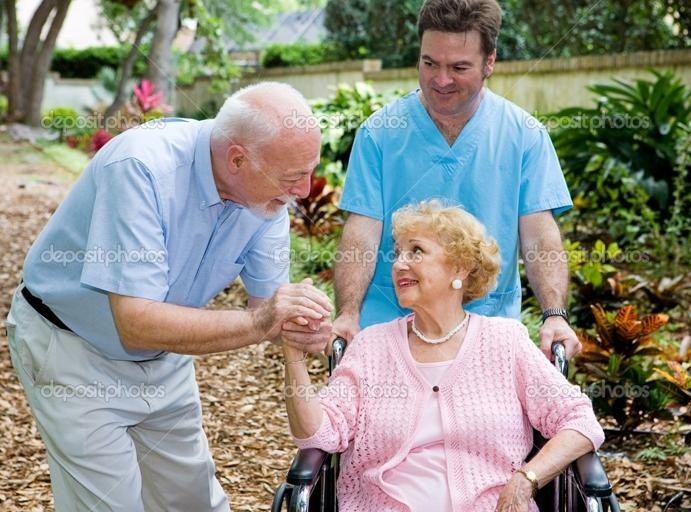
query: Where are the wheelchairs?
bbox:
[268,334,625,512]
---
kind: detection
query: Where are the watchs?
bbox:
[519,463,541,493]
[543,305,571,324]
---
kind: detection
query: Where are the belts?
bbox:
[20,278,75,334]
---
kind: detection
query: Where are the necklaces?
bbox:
[411,312,470,345]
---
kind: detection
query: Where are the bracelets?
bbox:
[284,355,309,368]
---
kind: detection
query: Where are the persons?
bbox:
[315,2,586,360]
[273,190,607,511]
[0,81,334,511]
